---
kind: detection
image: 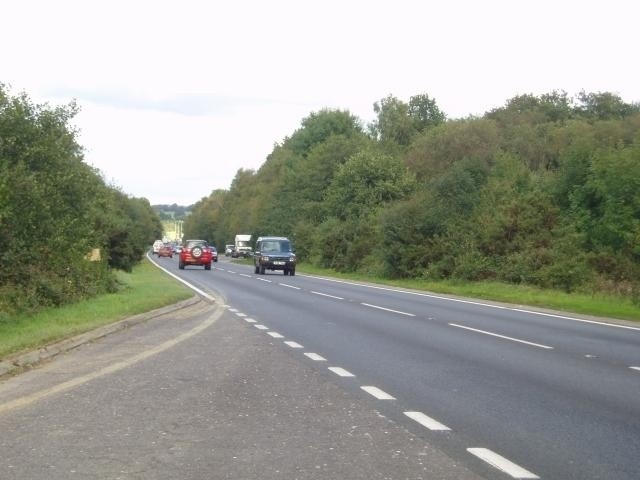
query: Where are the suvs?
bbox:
[251,234,297,277]
[178,239,212,271]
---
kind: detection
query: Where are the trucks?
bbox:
[232,233,253,258]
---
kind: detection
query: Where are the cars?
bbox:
[152,238,254,263]
